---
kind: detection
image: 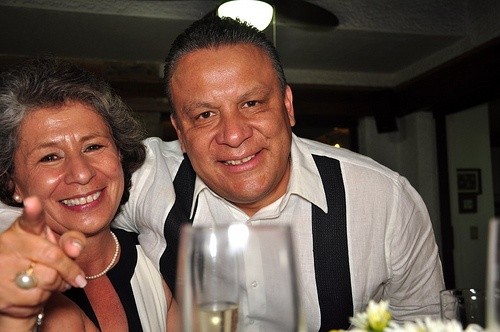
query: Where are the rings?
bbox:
[16,261,37,289]
[38,313,44,324]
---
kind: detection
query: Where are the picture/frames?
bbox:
[457,168,482,195]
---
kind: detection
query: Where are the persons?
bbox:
[0,58,174,332]
[0,14,450,332]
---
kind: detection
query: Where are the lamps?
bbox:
[218,0,274,32]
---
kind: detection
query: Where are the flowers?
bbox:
[330,299,484,332]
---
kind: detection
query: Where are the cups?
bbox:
[438,285,500,330]
[178,222,306,332]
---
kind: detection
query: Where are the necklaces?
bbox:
[85,230,119,279]
